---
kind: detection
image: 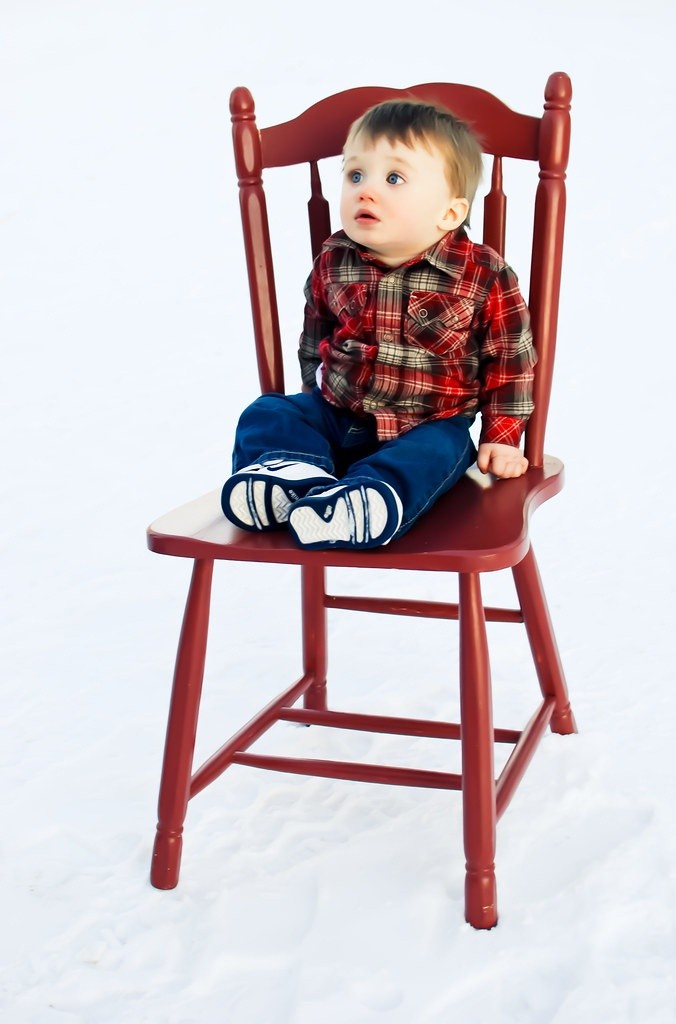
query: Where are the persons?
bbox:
[222,98,538,556]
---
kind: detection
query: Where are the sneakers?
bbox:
[287,476,403,550]
[221,458,339,533]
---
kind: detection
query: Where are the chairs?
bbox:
[144,71,578,930]
